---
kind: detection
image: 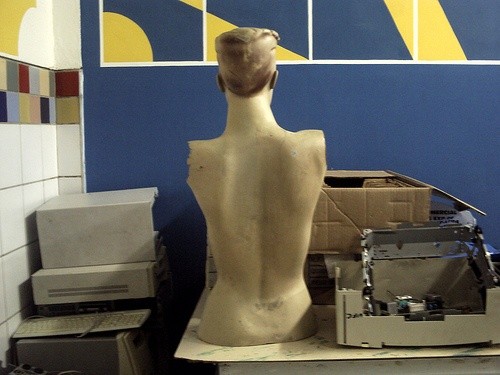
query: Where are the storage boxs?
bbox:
[308,170,487,255]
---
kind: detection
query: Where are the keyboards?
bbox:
[10,307,152,338]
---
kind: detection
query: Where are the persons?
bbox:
[186,27,327,347]
